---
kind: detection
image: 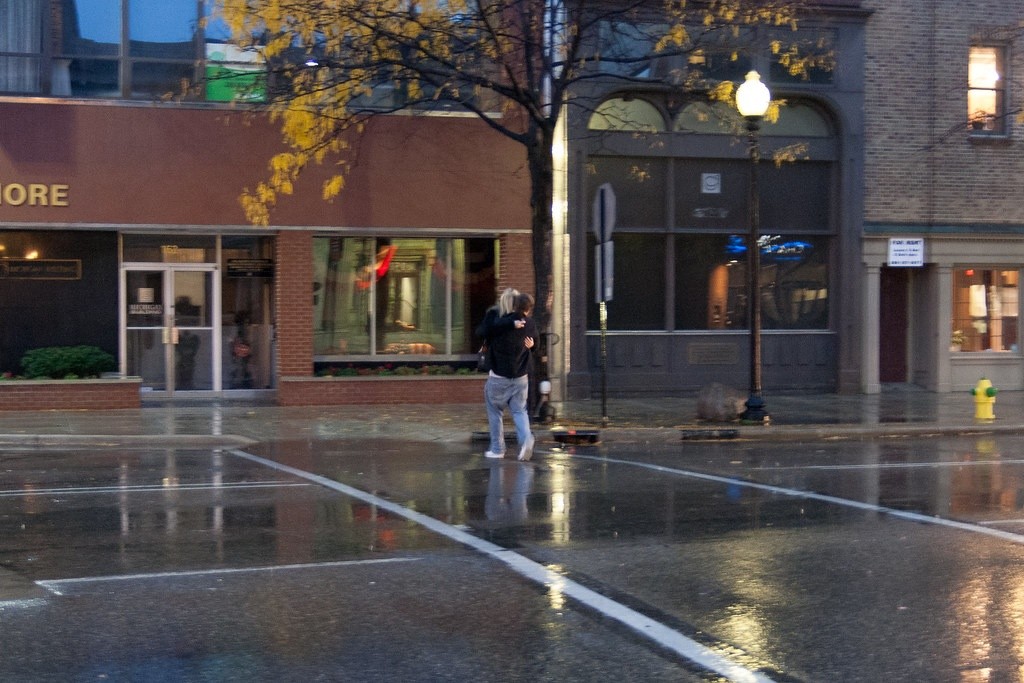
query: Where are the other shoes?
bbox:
[485,449,506,459]
[518,436,535,461]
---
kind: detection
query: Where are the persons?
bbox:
[474,287,541,460]
[176,330,201,389]
[482,459,533,548]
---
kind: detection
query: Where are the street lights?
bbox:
[736,69,770,424]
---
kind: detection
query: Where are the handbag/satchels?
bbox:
[477,341,492,373]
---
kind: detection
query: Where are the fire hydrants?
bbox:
[972,377,997,419]
